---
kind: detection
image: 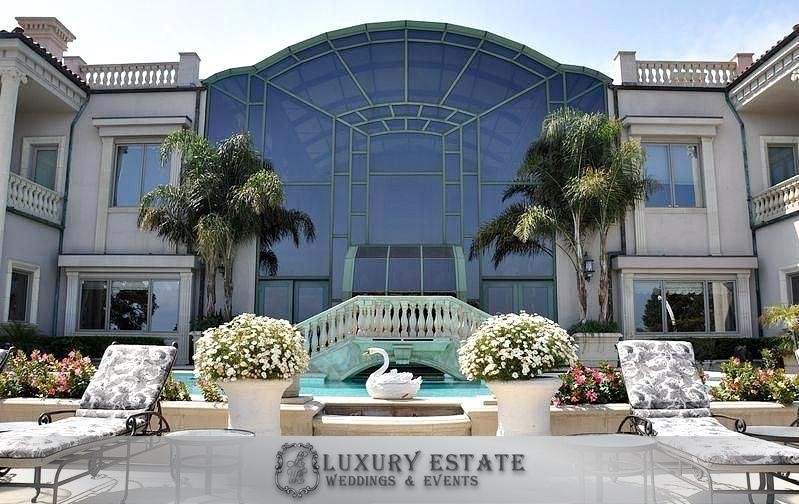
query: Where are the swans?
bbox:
[361,347,423,401]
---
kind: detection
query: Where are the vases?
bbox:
[217,378,291,438]
[484,376,564,438]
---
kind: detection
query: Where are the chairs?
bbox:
[612,335,799,504]
[0,343,177,503]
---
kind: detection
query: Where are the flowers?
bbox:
[184,312,311,384]
[456,311,580,388]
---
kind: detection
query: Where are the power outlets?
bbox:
[569,424,798,504]
